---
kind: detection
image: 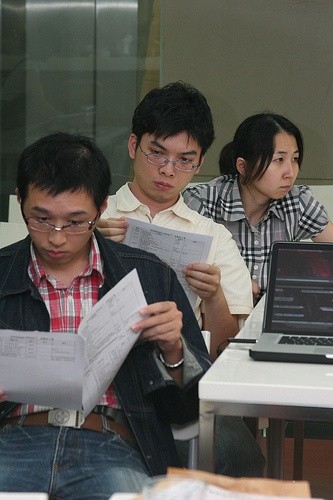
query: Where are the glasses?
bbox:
[20,198,100,234]
[137,137,201,172]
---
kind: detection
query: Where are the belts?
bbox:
[3,409,136,442]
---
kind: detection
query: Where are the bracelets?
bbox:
[160,353,184,368]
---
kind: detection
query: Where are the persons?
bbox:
[181,113,333,308]
[0,131,216,500]
[94,80,267,481]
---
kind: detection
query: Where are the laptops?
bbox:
[248,240,333,366]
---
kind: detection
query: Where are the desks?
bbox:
[198,292,333,479]
